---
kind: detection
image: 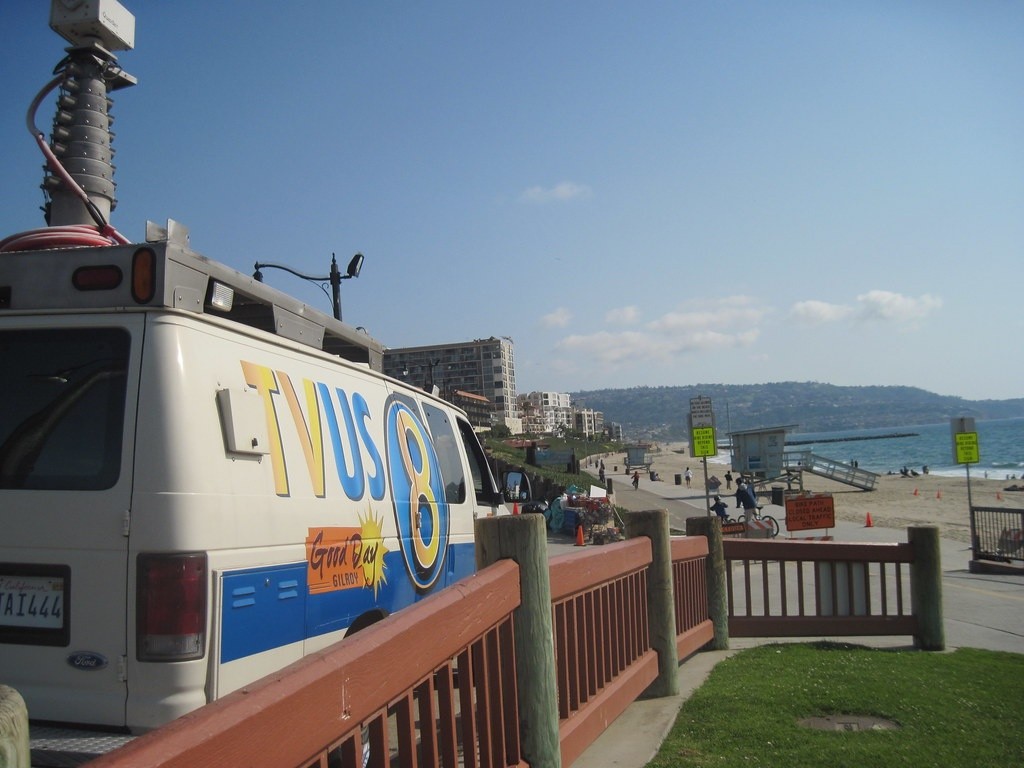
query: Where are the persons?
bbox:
[734,477,761,525]
[684,466,693,489]
[724,470,733,491]
[709,495,729,525]
[590,453,665,490]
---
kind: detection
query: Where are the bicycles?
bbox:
[599,474,605,483]
[721,514,737,525]
[632,476,639,489]
[736,505,780,537]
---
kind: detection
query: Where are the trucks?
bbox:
[0,218,535,768]
